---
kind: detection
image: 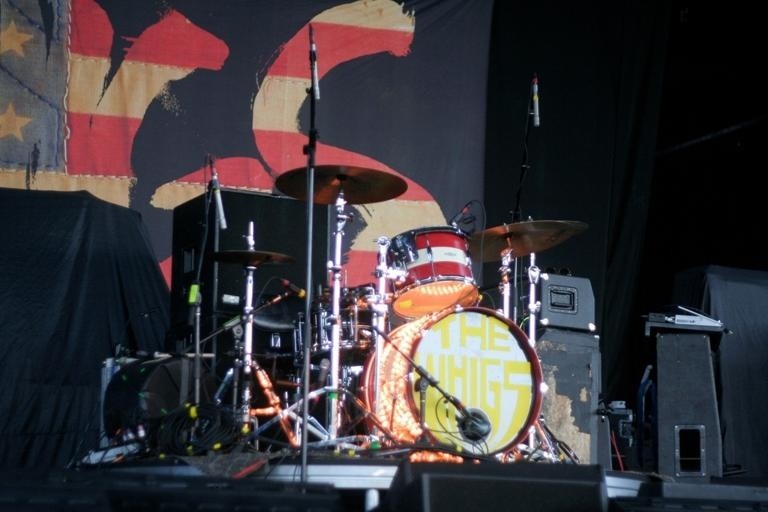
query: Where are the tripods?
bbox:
[201,265,300,450]
[234,193,402,452]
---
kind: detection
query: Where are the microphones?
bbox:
[209,155,228,230]
[448,393,491,444]
[450,201,474,227]
[309,29,321,99]
[532,75,540,127]
[280,278,306,299]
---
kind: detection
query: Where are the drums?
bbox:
[357,305,544,459]
[311,284,375,366]
[378,226,479,321]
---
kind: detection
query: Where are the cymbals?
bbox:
[206,251,298,265]
[275,165,408,205]
[469,220,588,262]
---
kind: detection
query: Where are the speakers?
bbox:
[481,273,599,332]
[174,193,329,312]
[533,329,600,465]
[104,356,194,440]
[637,332,725,479]
[391,474,608,512]
[386,462,604,498]
